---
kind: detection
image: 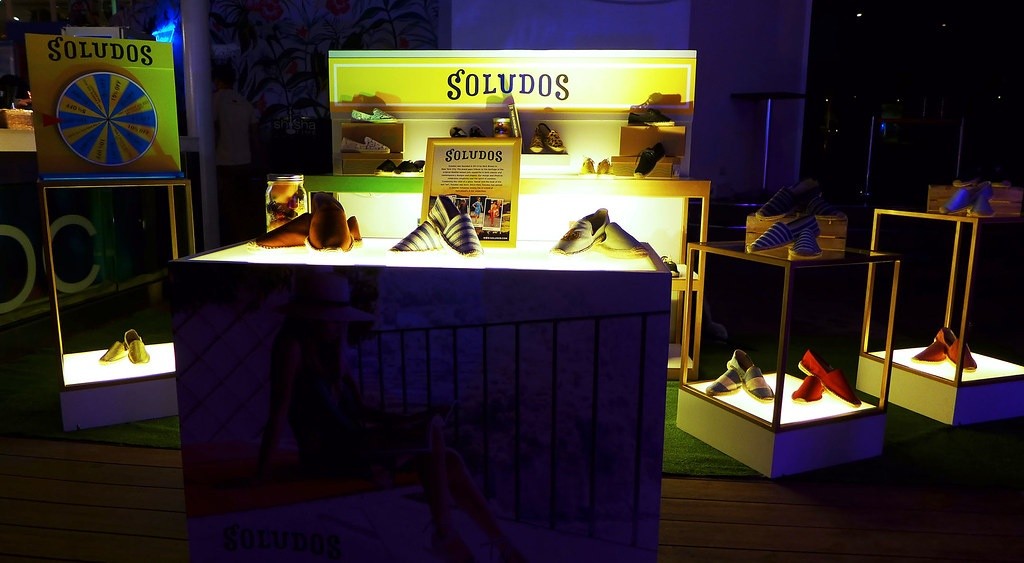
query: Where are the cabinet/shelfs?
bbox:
[43,178,197,432]
[856,206,1024,427]
[675,239,901,478]
[170,239,670,563]
[278,100,712,383]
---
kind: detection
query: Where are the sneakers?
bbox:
[393,159,425,176]
[581,157,612,175]
[98,340,128,364]
[914,325,978,374]
[374,159,396,174]
[660,255,682,278]
[745,213,823,260]
[124,328,152,366]
[627,107,675,127]
[938,180,994,220]
[791,348,862,409]
[256,211,362,249]
[528,126,545,154]
[352,107,397,123]
[552,207,611,257]
[427,192,486,257]
[568,217,649,261]
[388,220,448,252]
[707,349,774,404]
[537,122,567,153]
[755,175,848,221]
[339,134,390,155]
[634,141,667,179]
[305,190,354,253]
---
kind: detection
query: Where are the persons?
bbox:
[210,64,259,240]
